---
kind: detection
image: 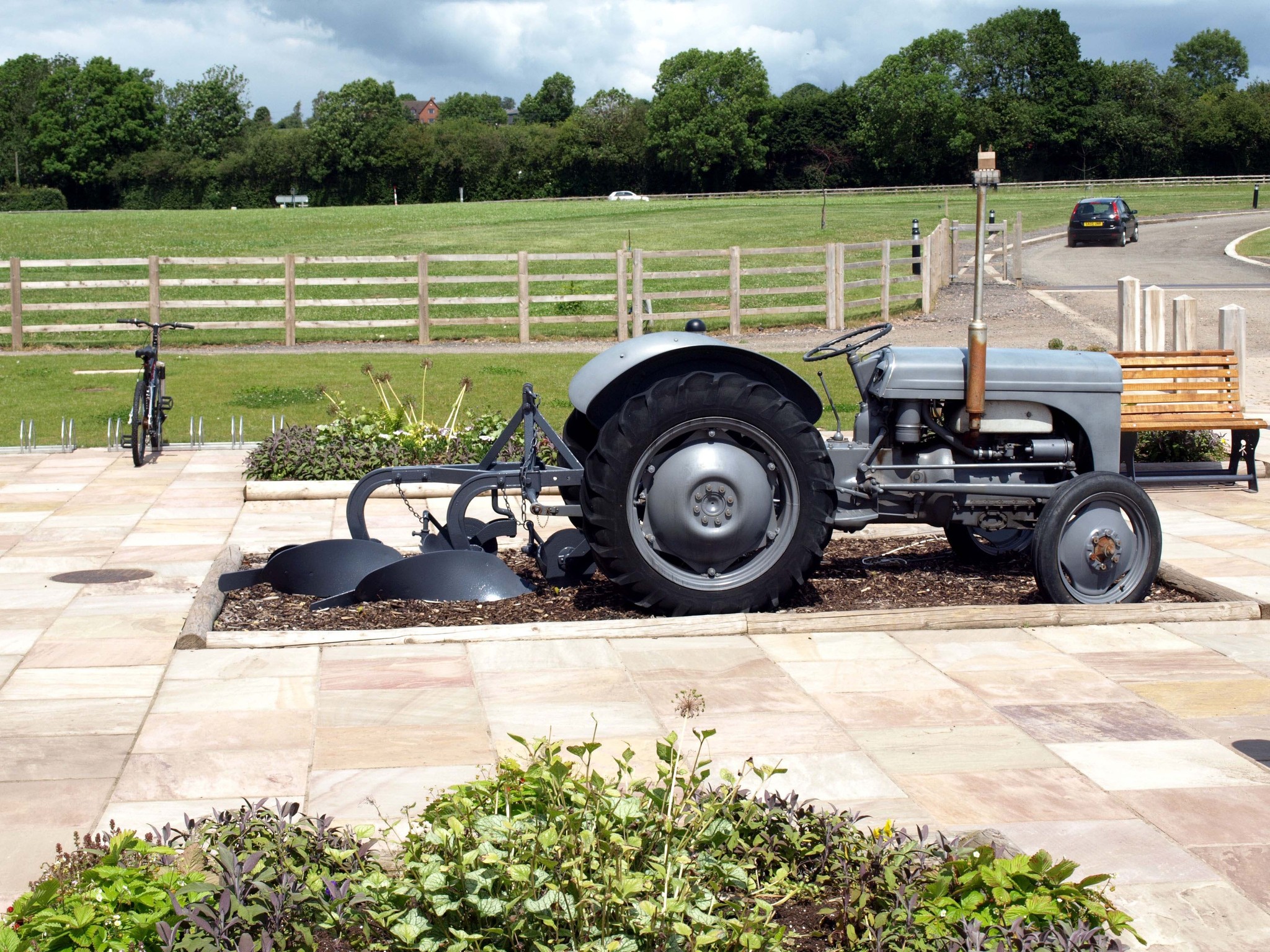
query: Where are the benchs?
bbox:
[1107,349,1270,491]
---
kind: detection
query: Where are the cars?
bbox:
[608,191,649,202]
[1067,195,1138,248]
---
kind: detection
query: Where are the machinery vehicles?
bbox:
[216,144,1168,616]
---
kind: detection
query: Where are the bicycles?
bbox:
[115,318,195,468]
